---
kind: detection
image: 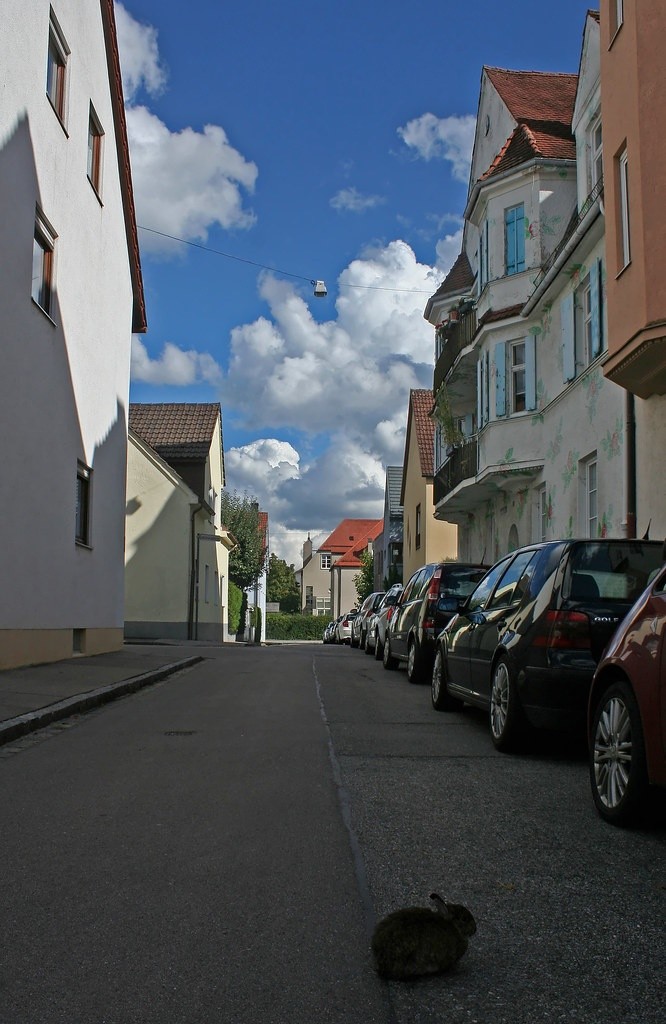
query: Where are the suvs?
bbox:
[382,561,496,684]
[432,537,666,756]
[365,583,406,660]
[350,589,386,652]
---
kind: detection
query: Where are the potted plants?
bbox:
[432,383,465,456]
[448,304,458,321]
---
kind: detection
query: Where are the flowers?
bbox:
[442,319,448,325]
[436,323,442,329]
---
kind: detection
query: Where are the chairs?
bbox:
[571,573,600,596]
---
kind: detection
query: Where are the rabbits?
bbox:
[371,893,476,982]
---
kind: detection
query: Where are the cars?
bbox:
[335,613,358,645]
[586,560,666,826]
[323,621,337,645]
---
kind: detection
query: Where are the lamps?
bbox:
[314,280,328,297]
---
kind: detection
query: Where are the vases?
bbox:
[444,322,448,326]
